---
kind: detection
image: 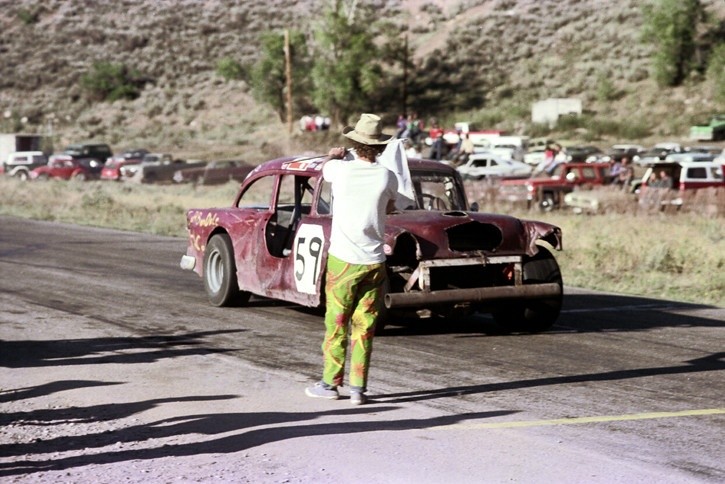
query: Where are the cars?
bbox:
[440,121,725,215]
[0,133,159,180]
[174,157,258,186]
[179,150,564,337]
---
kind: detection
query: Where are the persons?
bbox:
[446,122,470,169]
[397,114,421,139]
[659,171,673,207]
[301,115,331,131]
[524,139,567,212]
[429,116,443,160]
[305,113,398,405]
[638,172,661,203]
[609,154,634,194]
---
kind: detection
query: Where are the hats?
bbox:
[342,113,394,145]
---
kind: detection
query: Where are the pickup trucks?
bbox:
[118,152,209,184]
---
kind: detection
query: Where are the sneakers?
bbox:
[350,391,367,405]
[305,381,339,399]
